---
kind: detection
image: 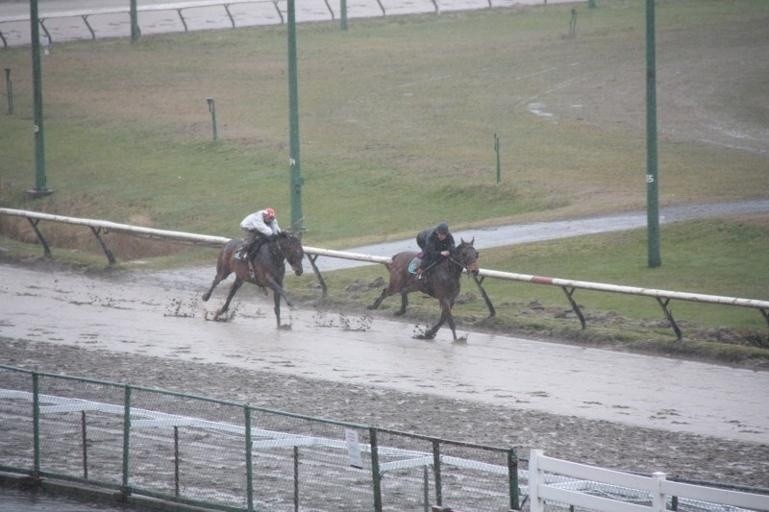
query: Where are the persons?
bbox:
[238,207,281,261]
[414,221,455,279]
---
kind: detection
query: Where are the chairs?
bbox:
[529,449,769,512]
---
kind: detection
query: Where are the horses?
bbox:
[201,231,304,329]
[367,237,479,340]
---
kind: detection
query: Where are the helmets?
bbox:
[263,208,274,219]
[438,223,448,235]
